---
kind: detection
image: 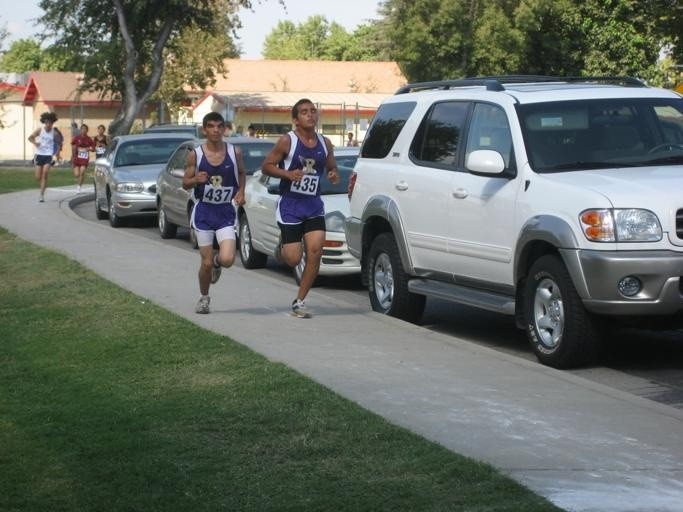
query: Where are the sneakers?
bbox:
[274,234,285,265]
[290,298,312,318]
[211,250,222,284]
[196,296,210,313]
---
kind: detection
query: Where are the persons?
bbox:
[261,99,339,320]
[71,120,106,192]
[347,132,358,147]
[248,127,255,139]
[184,112,245,314]
[225,121,236,136]
[27,111,60,202]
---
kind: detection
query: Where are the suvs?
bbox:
[343,71,683,372]
